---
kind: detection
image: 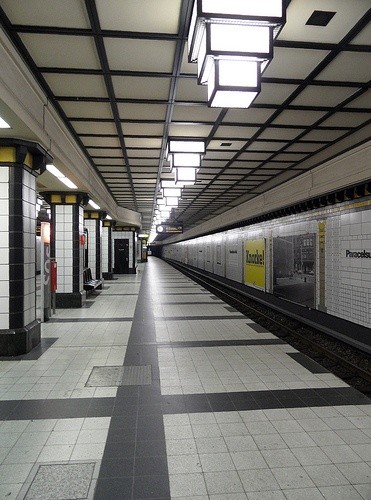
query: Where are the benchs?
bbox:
[83,267,102,298]
